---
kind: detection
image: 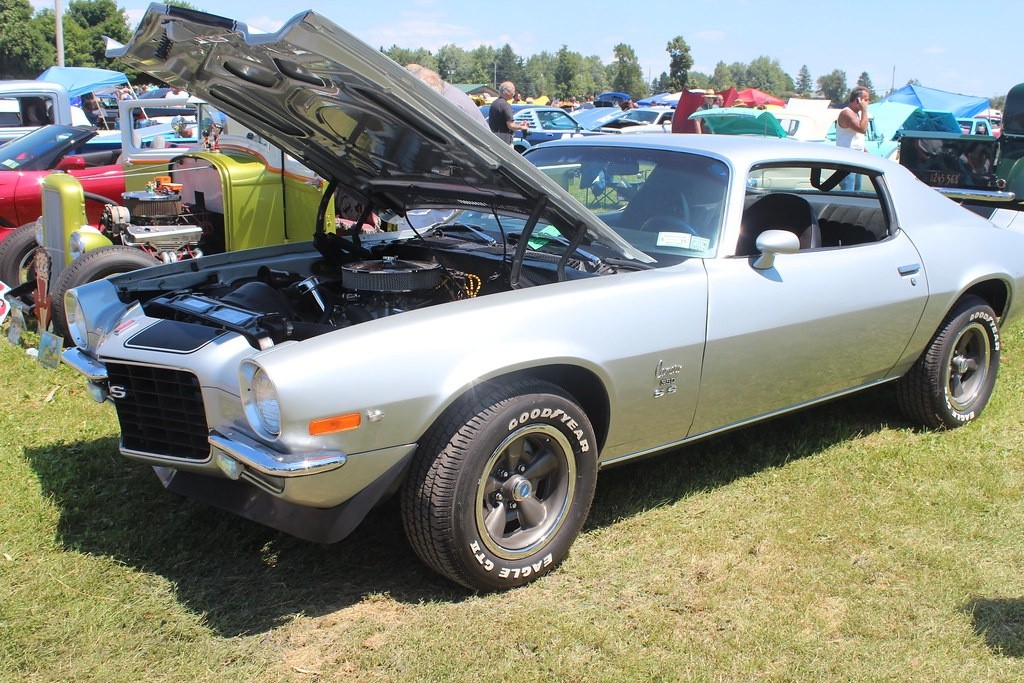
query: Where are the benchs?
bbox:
[744,195,889,243]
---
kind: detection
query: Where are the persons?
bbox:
[835,86,870,190]
[914,139,943,169]
[512,93,521,104]
[694,89,724,134]
[570,94,596,109]
[115,84,147,100]
[405,63,491,131]
[167,85,189,109]
[651,100,657,106]
[615,98,639,111]
[84,99,107,127]
[546,96,559,106]
[526,95,533,104]
[489,81,530,149]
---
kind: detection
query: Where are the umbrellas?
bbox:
[637,89,706,109]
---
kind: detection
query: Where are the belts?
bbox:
[494,130,512,135]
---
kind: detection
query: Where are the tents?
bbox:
[35,65,148,130]
[876,83,991,125]
[736,88,785,106]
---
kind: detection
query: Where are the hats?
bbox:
[526,97,534,103]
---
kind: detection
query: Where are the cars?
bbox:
[0,0,1023,354]
[56,9,1022,595]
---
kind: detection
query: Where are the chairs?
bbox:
[27,105,48,126]
[739,193,822,253]
[585,157,652,208]
